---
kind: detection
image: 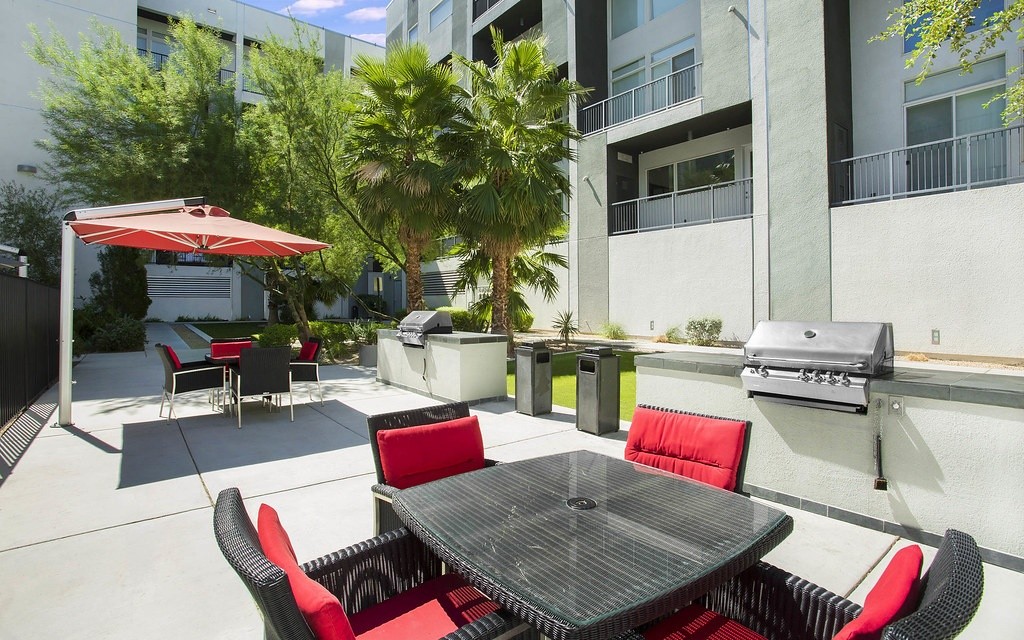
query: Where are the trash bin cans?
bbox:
[576,346,621,435]
[515,341,553,415]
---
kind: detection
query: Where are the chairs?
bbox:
[155,343,226,424]
[215,487,541,640]
[278,337,324,412]
[211,337,251,357]
[625,405,752,500]
[367,400,504,538]
[609,528,985,640]
[229,346,294,428]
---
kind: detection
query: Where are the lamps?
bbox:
[17,165,36,173]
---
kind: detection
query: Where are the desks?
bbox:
[204,352,296,362]
[392,450,795,640]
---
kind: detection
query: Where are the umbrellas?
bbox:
[58,197,331,424]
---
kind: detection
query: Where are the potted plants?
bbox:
[358,330,378,366]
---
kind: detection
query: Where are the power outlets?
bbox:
[932,330,940,345]
